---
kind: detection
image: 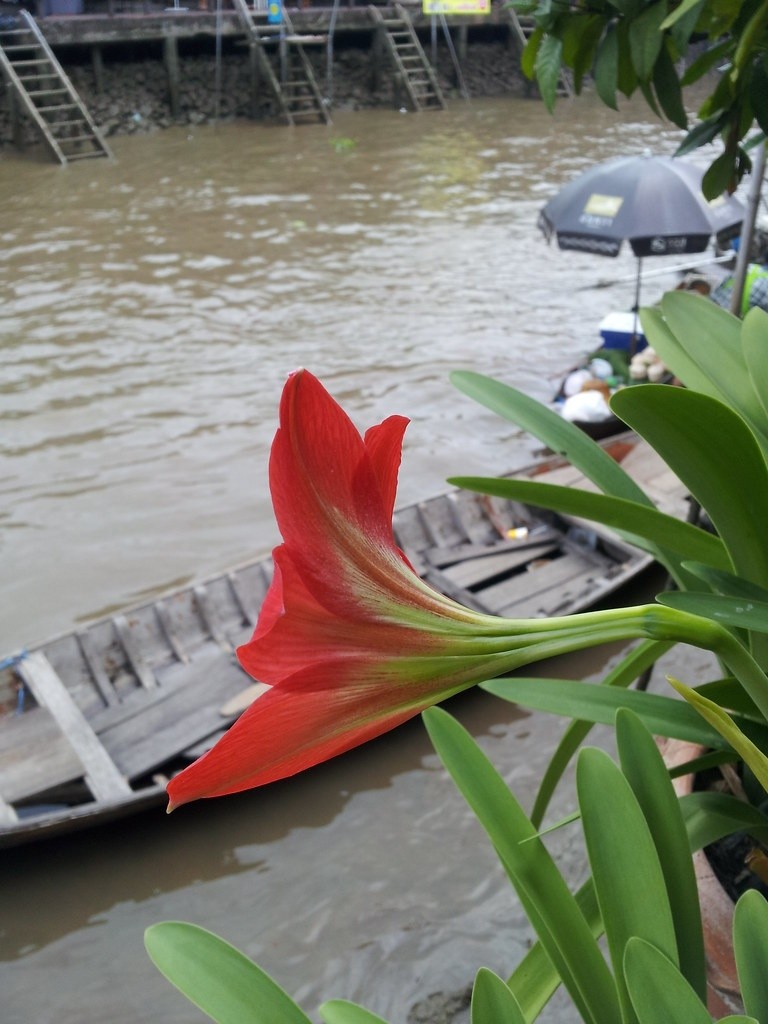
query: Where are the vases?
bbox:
[627,712,768,1024]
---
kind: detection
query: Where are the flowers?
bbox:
[144,287,768,1023]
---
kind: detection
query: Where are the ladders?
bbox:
[369,2,447,114]
[234,0,334,128]
[0,8,116,167]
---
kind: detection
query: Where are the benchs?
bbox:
[524,460,692,558]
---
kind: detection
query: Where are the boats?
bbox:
[1,431,707,852]
[529,302,677,459]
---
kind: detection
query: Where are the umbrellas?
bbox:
[537,153,748,346]
